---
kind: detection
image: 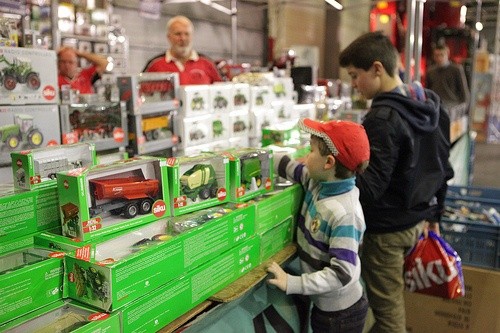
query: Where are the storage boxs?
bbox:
[0,1,500,333]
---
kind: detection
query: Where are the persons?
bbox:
[336,28,457,333]
[139,14,224,86]
[261,117,371,333]
[423,36,471,105]
[57,45,109,94]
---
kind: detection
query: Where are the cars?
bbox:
[234,90,247,106]
[191,91,205,109]
[233,120,247,133]
[214,91,229,109]
[211,120,224,137]
[189,127,206,140]
[241,158,264,190]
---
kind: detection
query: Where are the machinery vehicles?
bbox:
[0,55,42,90]
[73,262,107,299]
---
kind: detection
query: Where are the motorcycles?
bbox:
[1,114,44,149]
[181,164,220,202]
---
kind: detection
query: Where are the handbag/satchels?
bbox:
[401,231,465,299]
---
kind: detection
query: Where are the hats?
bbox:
[297,118,370,174]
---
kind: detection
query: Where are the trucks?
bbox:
[34,155,78,180]
[87,178,160,218]
[142,115,169,141]
